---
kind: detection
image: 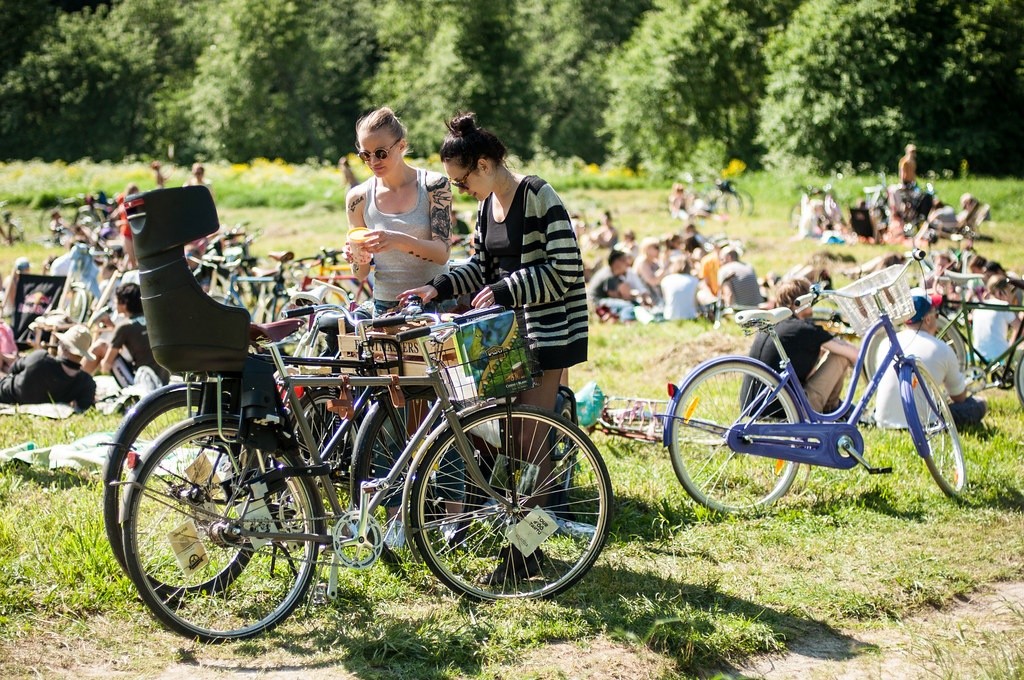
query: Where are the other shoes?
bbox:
[826,400,856,420]
[385,519,407,548]
[440,520,459,544]
[481,545,544,586]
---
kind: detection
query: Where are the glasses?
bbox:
[449,169,474,190]
[359,141,397,161]
[921,312,938,320]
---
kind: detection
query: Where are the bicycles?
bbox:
[666,174,755,223]
[859,268,1024,415]
[650,246,969,519]
[37,191,615,647]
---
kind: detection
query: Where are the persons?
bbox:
[877,296,985,428]
[395,118,588,583]
[669,184,712,219]
[91,186,139,312]
[49,211,90,277]
[342,108,462,547]
[0,257,33,318]
[740,278,860,420]
[887,145,977,243]
[183,163,215,197]
[81,283,170,389]
[0,326,97,411]
[152,162,167,189]
[804,199,823,238]
[571,212,765,323]
[185,247,212,292]
[339,158,359,190]
[451,211,476,257]
[928,240,1024,366]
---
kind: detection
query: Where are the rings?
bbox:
[488,300,492,304]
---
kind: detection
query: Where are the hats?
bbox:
[52,324,97,361]
[905,292,943,325]
[905,144,917,152]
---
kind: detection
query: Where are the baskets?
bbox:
[432,338,538,410]
[830,264,916,338]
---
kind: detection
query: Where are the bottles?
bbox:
[2,441,38,459]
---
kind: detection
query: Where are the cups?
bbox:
[346,227,373,265]
